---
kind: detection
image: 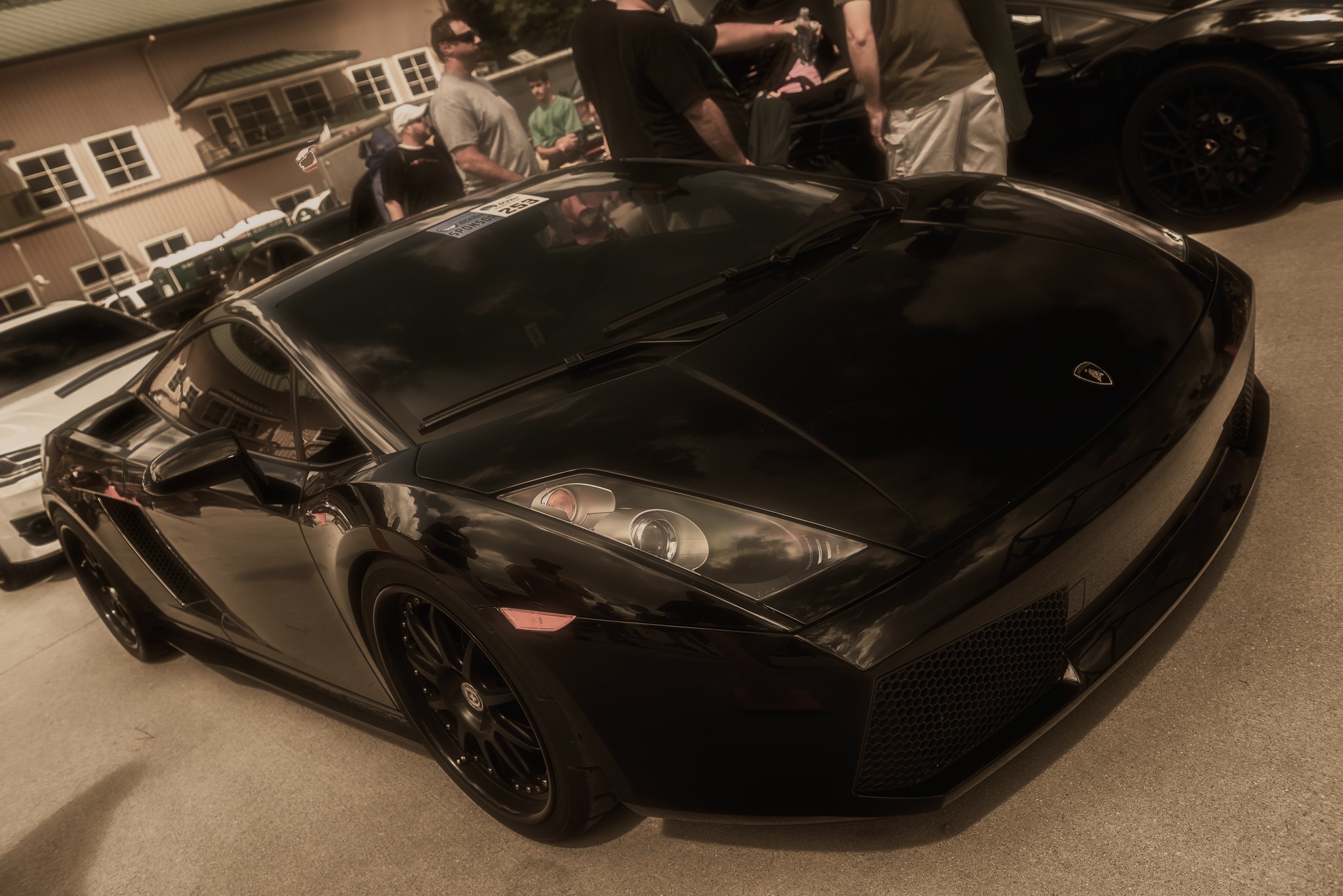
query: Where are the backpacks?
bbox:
[370,146,410,226]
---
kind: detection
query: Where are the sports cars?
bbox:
[783,1,1337,218]
[37,158,1272,841]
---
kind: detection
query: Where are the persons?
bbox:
[833,0,1011,183]
[526,65,589,171]
[568,1,824,174]
[356,126,401,225]
[515,191,646,256]
[427,10,543,197]
[379,102,466,222]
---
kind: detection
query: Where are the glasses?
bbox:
[441,28,480,43]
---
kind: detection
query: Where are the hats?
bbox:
[390,102,429,135]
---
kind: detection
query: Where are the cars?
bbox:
[104,207,369,321]
[0,299,178,589]
